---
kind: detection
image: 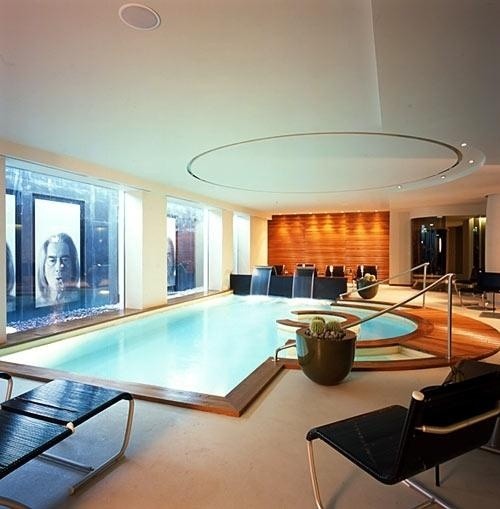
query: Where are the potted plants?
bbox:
[296,316,357,386]
[357,273,379,299]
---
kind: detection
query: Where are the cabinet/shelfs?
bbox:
[230,274,348,298]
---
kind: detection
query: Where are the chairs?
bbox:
[0,373,135,509]
[325,265,345,277]
[352,264,378,281]
[297,263,317,276]
[458,272,488,306]
[306,371,500,509]
[268,264,288,275]
[441,358,500,455]
[455,268,483,297]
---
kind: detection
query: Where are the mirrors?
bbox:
[418,229,448,276]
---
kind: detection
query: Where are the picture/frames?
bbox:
[168,216,177,288]
[32,192,84,309]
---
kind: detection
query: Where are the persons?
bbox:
[167,238,176,288]
[38,234,80,305]
